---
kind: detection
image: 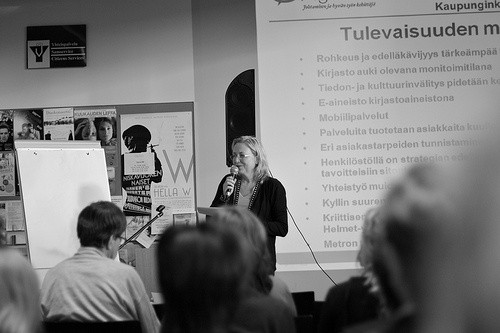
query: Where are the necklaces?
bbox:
[234,177,260,211]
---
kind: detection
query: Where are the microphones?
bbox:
[226,165,238,197]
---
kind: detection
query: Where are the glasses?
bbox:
[230,154,255,160]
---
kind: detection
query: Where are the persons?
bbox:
[0,157,500,333]
[205,135,288,276]
[0,117,118,151]
